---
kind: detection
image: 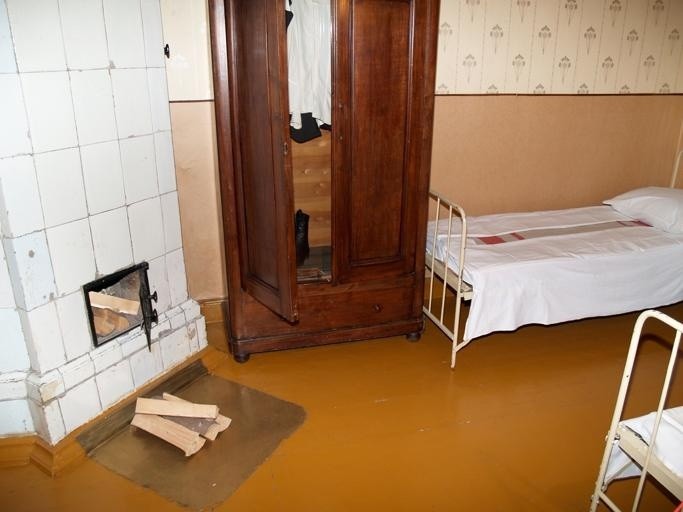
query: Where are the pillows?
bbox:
[603,185,683,239]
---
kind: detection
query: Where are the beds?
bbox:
[587,311,683,512]
[417,161,683,369]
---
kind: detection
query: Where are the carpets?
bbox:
[90,370,306,510]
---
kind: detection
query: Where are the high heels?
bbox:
[294,209,311,268]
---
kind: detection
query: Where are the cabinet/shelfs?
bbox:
[203,0,440,364]
